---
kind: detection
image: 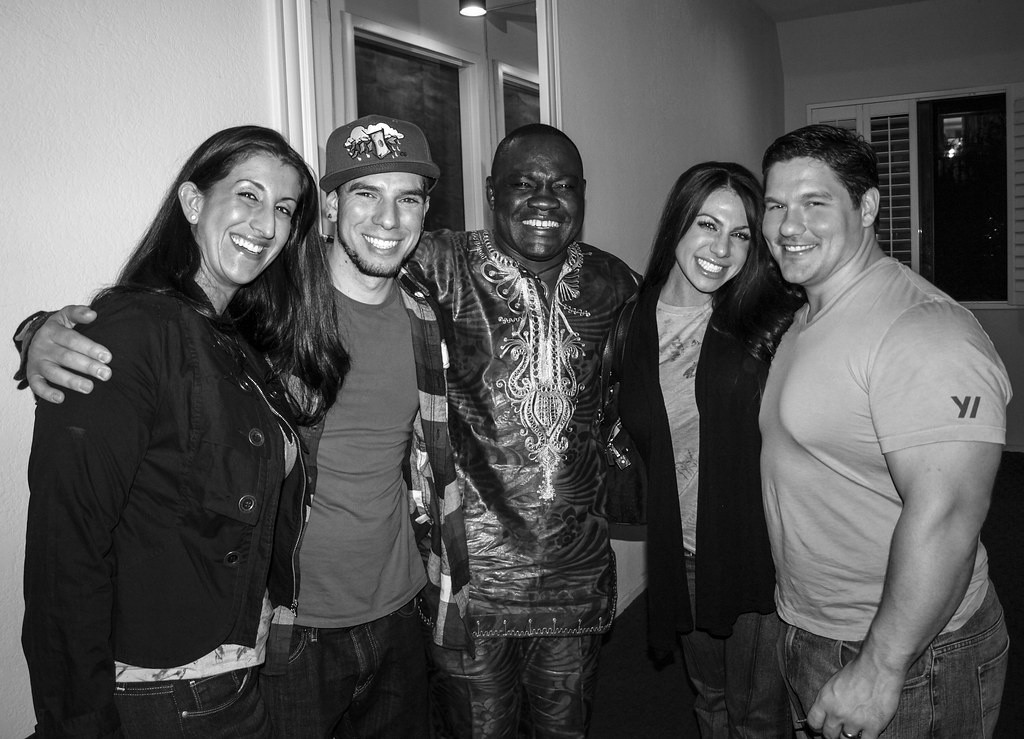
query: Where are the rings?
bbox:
[841,730,855,739]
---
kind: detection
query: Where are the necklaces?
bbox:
[496,240,566,304]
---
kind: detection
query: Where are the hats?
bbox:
[319,114,441,194]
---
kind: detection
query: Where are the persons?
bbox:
[604,161,792,739]
[401,124,643,739]
[11,113,476,739]
[20,125,350,739]
[757,125,1012,739]
